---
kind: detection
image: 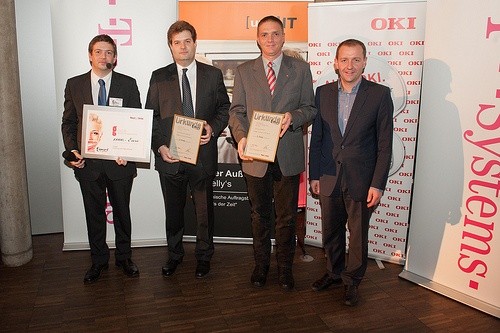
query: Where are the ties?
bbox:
[97,79,108,108]
[182,68,194,119]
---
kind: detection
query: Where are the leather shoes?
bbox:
[115,259,139,277]
[343,284,359,307]
[162,256,183,278]
[277,266,294,290]
[311,273,343,291]
[84,260,109,284]
[251,262,270,288]
[195,260,210,280]
[266,61,276,96]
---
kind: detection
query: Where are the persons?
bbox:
[308,39,393,306]
[61,34,142,285]
[228,16,318,288]
[143,20,231,278]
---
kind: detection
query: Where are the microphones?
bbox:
[106,62,114,69]
[62,151,87,167]
